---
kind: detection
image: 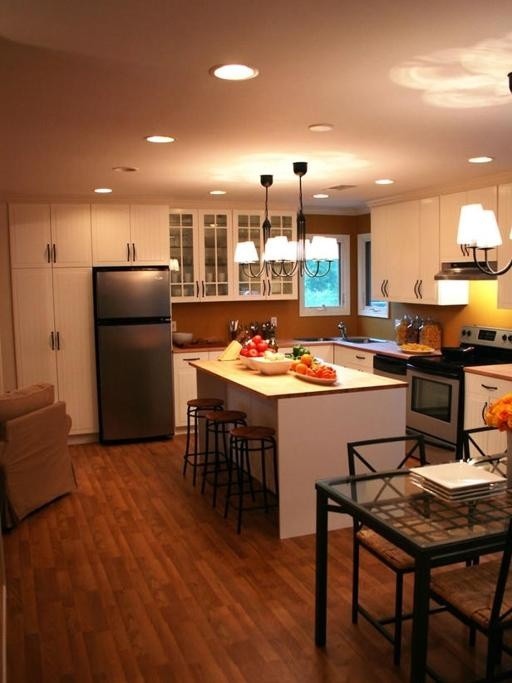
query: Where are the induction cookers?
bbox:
[412,349,508,375]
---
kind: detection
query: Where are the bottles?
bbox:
[394,314,442,350]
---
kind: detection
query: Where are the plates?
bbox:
[291,370,337,384]
[407,462,508,505]
[396,346,435,354]
[207,287,223,295]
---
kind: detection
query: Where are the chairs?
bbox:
[423,531,511,683]
[462,425,511,565]
[346,434,473,667]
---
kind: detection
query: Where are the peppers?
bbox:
[286,345,309,360]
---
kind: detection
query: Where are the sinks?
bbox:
[293,336,338,342]
[341,336,390,344]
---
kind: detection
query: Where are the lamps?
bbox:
[233,163,339,277]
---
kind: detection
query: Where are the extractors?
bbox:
[434,261,497,279]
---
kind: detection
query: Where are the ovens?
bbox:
[405,364,461,453]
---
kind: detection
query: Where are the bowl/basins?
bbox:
[172,332,192,346]
[246,356,293,376]
[238,353,262,370]
[171,286,188,296]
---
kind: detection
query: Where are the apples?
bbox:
[239,335,275,355]
[261,350,284,361]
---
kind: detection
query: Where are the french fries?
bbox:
[400,342,432,350]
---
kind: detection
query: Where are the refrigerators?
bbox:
[92,266,177,448]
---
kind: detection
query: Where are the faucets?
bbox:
[337,320,349,341]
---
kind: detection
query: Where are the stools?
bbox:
[183,398,277,535]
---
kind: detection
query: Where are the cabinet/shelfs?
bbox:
[370,196,469,305]
[172,343,333,428]
[90,201,169,268]
[334,342,375,374]
[8,199,97,444]
[233,209,297,300]
[463,365,512,458]
[167,206,234,301]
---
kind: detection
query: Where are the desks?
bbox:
[314,451,512,683]
[187,357,409,538]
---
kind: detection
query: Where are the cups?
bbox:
[207,273,214,283]
[184,272,191,283]
[172,271,178,283]
[170,235,177,246]
[218,272,226,282]
